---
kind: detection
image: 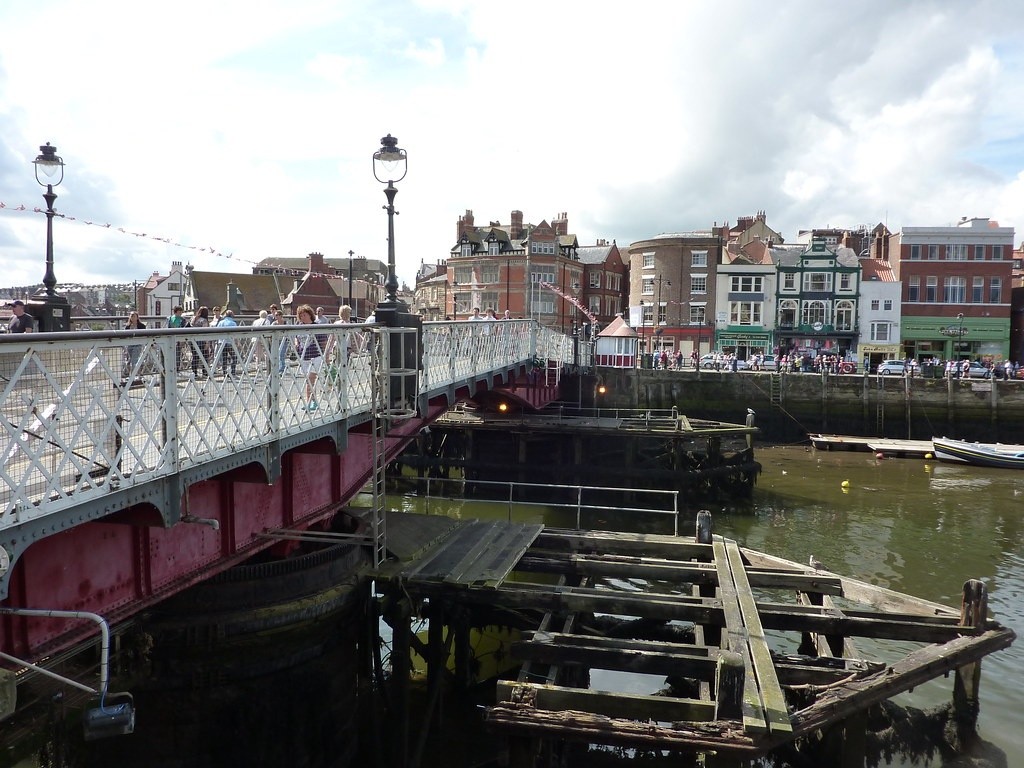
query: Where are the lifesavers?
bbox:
[843,364,852,374]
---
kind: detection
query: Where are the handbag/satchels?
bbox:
[290,346,299,361]
[183,316,194,340]
[352,332,367,354]
[162,316,171,328]
[324,366,339,386]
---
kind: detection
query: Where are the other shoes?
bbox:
[301,405,306,410]
[310,400,317,409]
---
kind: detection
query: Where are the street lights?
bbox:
[347,250,355,310]
[31,141,69,302]
[370,134,412,310]
[649,274,673,350]
[639,297,654,354]
[450,280,461,322]
[569,282,583,333]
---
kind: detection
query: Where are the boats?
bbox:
[930,436,1024,469]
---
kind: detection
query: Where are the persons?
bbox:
[654,347,1021,380]
[5,297,524,412]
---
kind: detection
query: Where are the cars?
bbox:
[877,358,921,375]
[698,353,747,370]
[756,354,782,371]
[950,361,992,379]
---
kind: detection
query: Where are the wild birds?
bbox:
[809,555,829,575]
[747,408,756,414]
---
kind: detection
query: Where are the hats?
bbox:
[8,300,24,307]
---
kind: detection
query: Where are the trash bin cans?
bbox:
[921,362,934,377]
[643,353,654,369]
[933,365,944,379]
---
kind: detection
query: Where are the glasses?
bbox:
[214,310,219,312]
